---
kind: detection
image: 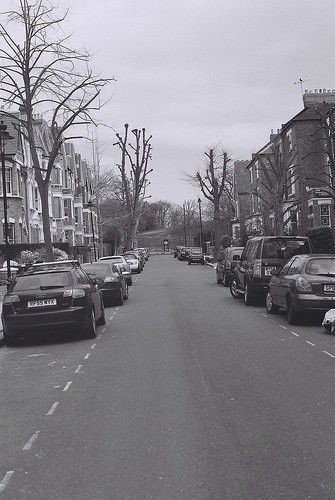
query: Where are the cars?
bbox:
[173,246,204,265]
[99,256,132,285]
[216,246,245,287]
[121,247,150,274]
[81,261,129,306]
[265,254,335,324]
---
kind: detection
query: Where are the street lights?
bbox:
[0,120,14,291]
[198,198,204,247]
[87,199,97,262]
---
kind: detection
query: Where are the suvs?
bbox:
[229,235,313,305]
[2,259,105,346]
